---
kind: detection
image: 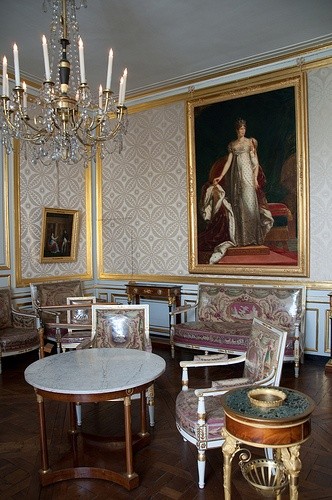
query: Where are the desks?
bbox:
[125,285,182,350]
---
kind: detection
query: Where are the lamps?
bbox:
[0,0,129,168]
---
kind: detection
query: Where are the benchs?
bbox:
[169,282,307,378]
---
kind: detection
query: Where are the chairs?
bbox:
[0,287,45,359]
[60,295,96,352]
[76,303,155,427]
[175,317,287,489]
[29,280,105,354]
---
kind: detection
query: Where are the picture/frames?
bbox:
[184,72,310,278]
[40,207,81,263]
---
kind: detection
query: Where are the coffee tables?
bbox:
[24,347,167,491]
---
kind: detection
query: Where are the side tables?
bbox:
[221,385,315,500]
